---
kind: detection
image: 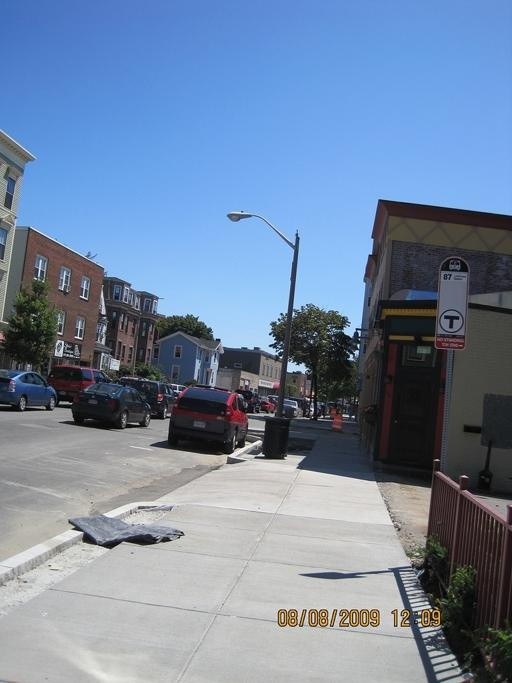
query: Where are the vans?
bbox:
[118,377,177,419]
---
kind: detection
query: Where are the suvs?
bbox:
[46,364,112,407]
[168,387,248,454]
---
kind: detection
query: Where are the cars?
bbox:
[1,368,58,410]
[72,382,151,429]
[166,383,346,418]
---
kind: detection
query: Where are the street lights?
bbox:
[226,209,300,459]
[130,312,164,377]
